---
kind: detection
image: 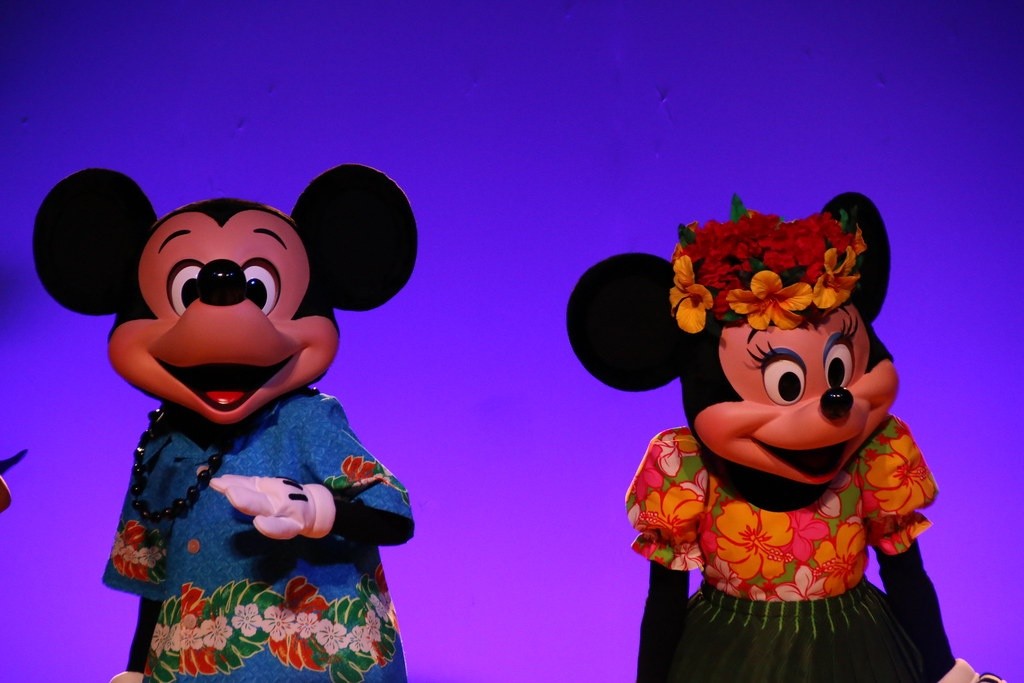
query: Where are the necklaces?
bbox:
[131,410,254,522]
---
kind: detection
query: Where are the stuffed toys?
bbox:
[566,193,1013,683]
[31,162,419,683]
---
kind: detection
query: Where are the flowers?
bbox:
[664,192,870,335]
